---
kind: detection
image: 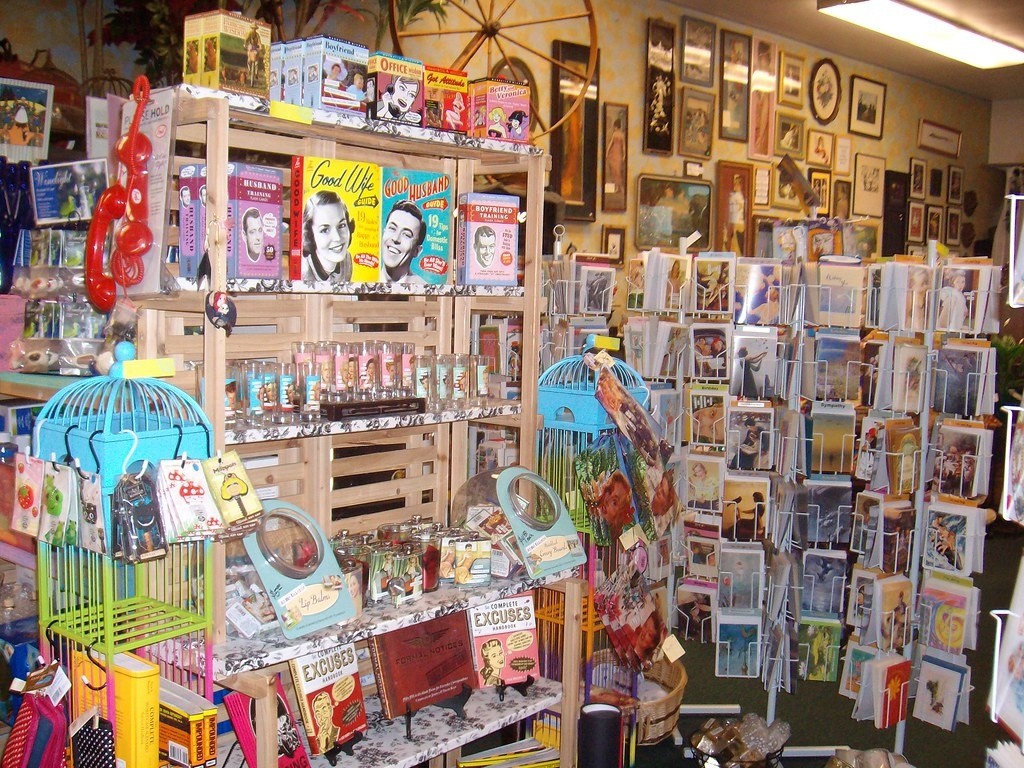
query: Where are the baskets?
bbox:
[580,647,687,749]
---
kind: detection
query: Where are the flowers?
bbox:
[83,0,246,88]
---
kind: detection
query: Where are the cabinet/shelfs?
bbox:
[530,334,651,750]
[136,98,586,768]
[33,342,217,768]
[0,371,134,579]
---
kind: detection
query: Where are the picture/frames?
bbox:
[491,13,966,270]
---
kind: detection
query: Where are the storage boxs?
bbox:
[0,616,69,728]
